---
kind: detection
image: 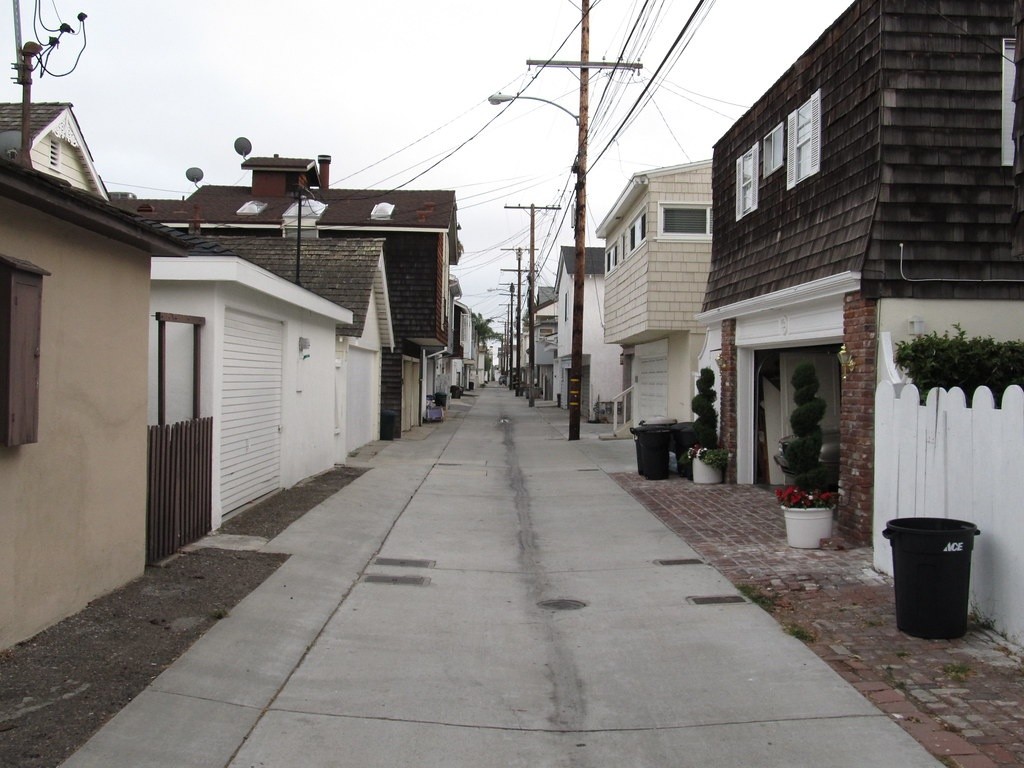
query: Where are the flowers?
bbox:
[775,485,840,510]
[688,445,707,459]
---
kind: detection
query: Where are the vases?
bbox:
[692,457,724,485]
[781,505,834,549]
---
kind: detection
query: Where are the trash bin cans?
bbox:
[381,409,395,441]
[435,391,448,411]
[629,417,698,481]
[449,384,464,399]
[881,517,981,640]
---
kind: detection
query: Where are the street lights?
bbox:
[490,0,642,442]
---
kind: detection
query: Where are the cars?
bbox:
[499,375,506,385]
